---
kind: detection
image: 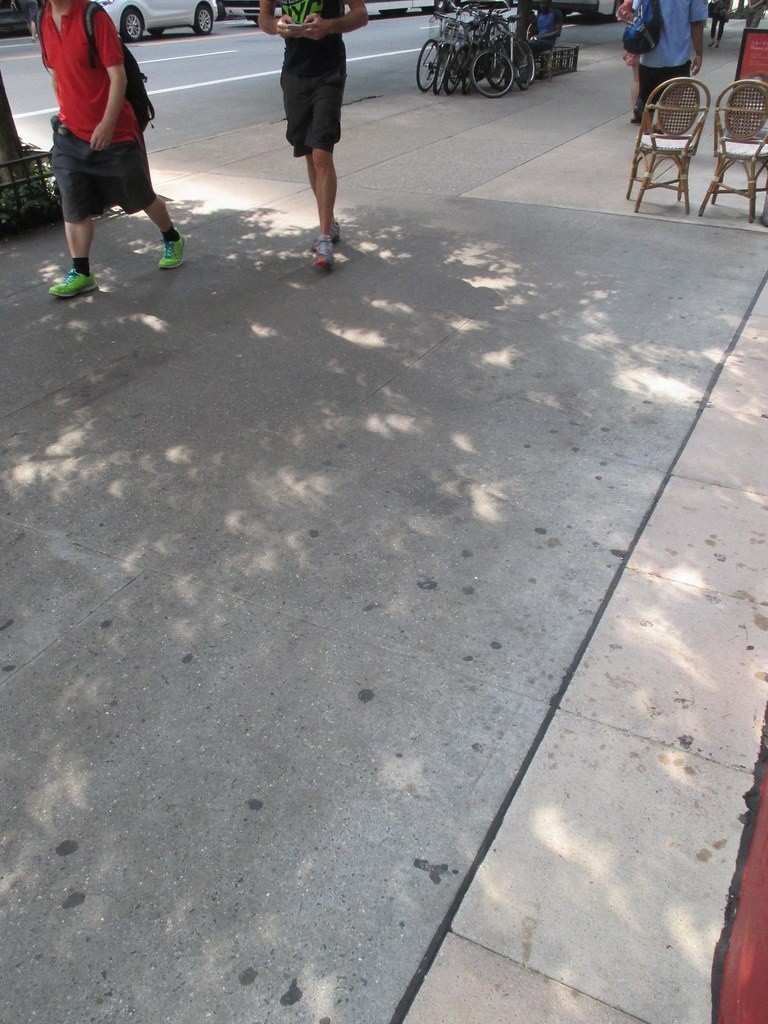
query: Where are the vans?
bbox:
[223,0,446,26]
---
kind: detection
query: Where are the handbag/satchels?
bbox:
[708,0,722,18]
[622,0,661,54]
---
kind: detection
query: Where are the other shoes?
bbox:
[652,125,664,135]
[32,34,39,43]
[715,44,719,48]
[631,107,642,123]
[708,43,714,47]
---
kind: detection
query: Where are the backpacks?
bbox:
[36,1,155,132]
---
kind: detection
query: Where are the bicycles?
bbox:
[414,0,536,100]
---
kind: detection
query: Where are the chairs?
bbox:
[526,24,556,81]
[697,79,768,224]
[625,76,710,213]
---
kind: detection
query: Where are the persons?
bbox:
[256,0,370,271]
[11,0,45,42]
[523,0,564,84]
[34,0,188,298]
[615,0,768,127]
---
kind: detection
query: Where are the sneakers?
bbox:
[48,267,97,297]
[158,230,185,268]
[311,221,340,252]
[315,238,334,266]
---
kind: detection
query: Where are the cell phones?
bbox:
[288,24,302,31]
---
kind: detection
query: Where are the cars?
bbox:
[87,0,220,45]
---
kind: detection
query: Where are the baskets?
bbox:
[428,16,468,47]
